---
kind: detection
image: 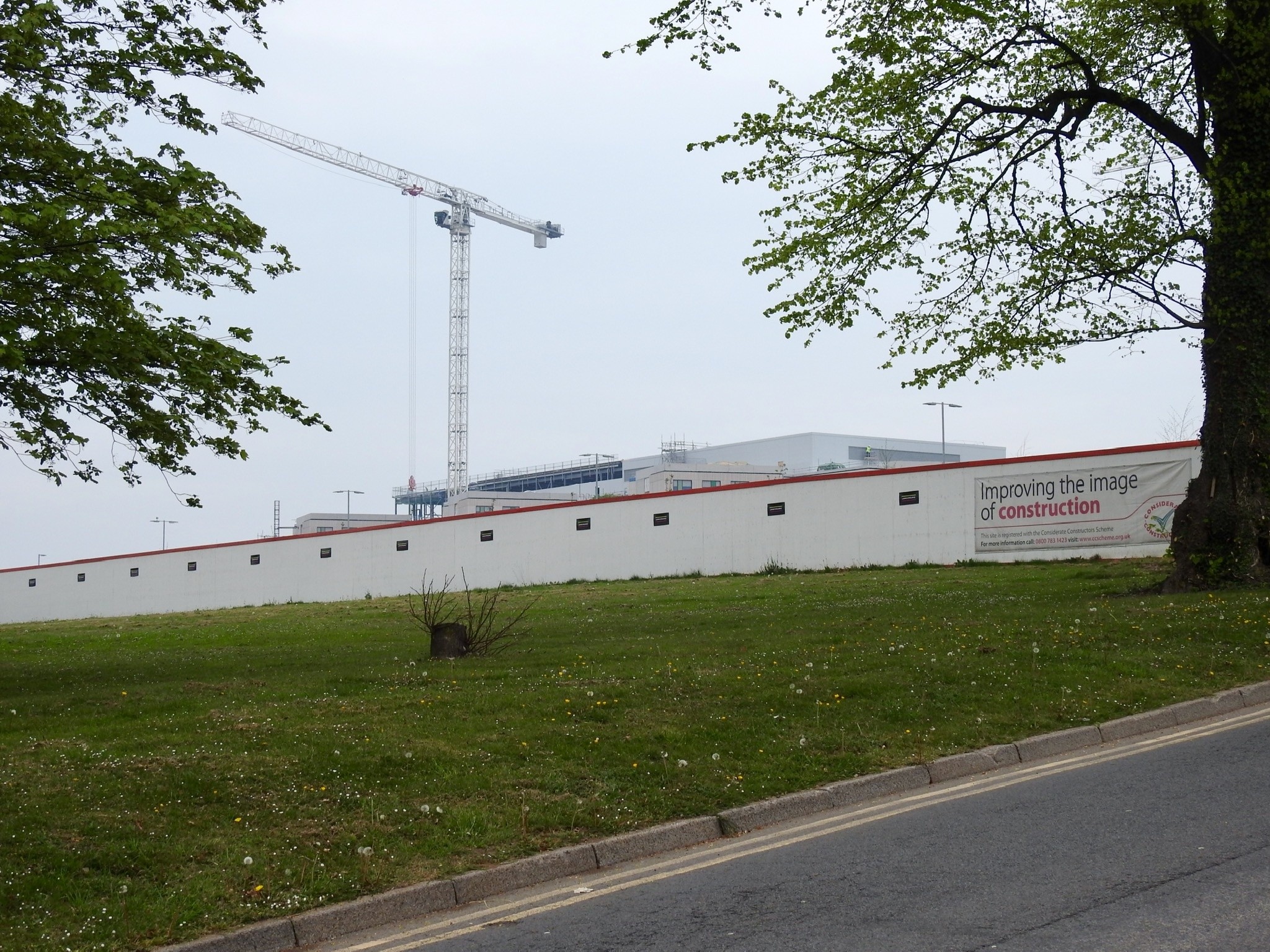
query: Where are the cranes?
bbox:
[219,109,566,501]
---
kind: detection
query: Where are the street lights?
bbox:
[38,554,46,565]
[332,490,365,530]
[578,453,615,499]
[922,402,963,464]
[150,520,179,550]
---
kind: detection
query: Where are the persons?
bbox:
[866,445,871,457]
